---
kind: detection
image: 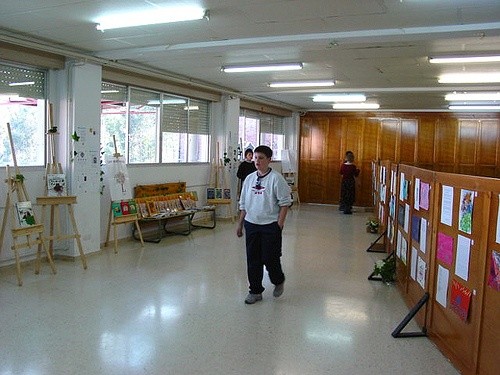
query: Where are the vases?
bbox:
[370,226,377,233]
[380,271,395,281]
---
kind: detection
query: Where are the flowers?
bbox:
[367,217,380,229]
[372,257,395,274]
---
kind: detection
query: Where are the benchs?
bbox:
[130,191,216,244]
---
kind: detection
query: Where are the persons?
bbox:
[237,148,258,222]
[236,145,293,304]
[339,151,360,214]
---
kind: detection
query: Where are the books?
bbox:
[207,187,231,200]
[136,198,215,219]
[15,201,36,226]
[286,177,294,187]
[46,173,67,196]
[112,199,138,217]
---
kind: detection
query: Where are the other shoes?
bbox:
[350,208,358,212]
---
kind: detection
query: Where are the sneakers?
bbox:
[244,293,262,304]
[273,281,284,297]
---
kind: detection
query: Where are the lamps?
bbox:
[428,54,500,63]
[94,8,210,32]
[220,62,304,73]
[266,80,337,89]
